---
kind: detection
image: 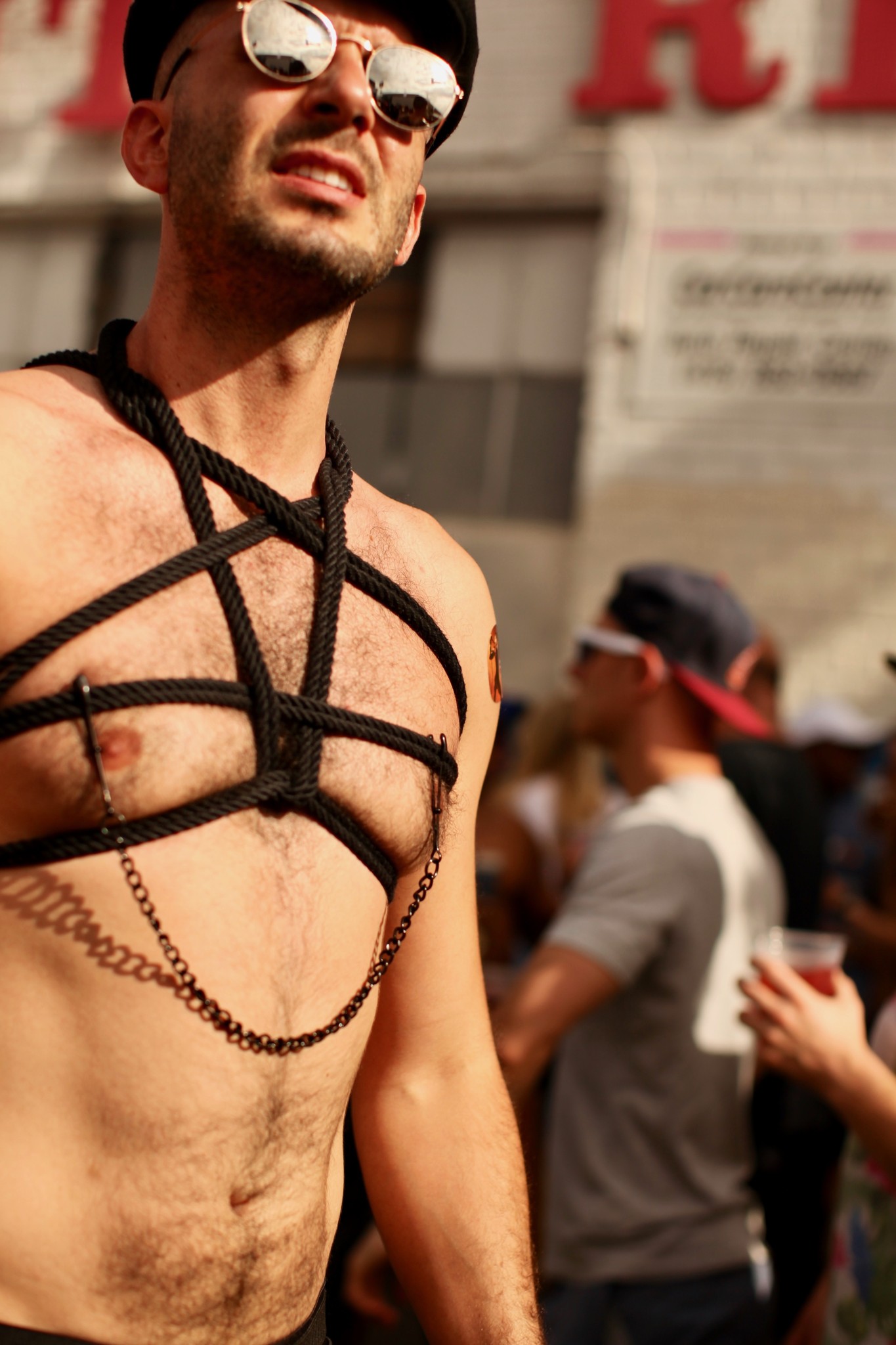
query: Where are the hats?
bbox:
[119,0,479,162]
[608,563,776,743]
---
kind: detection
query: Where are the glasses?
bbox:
[568,620,642,674]
[153,0,463,159]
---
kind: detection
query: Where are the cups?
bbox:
[762,926,847,1003]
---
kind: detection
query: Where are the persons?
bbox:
[0,0,545,1345]
[345,560,779,1345]
[379,632,892,1264]
[727,948,895,1345]
